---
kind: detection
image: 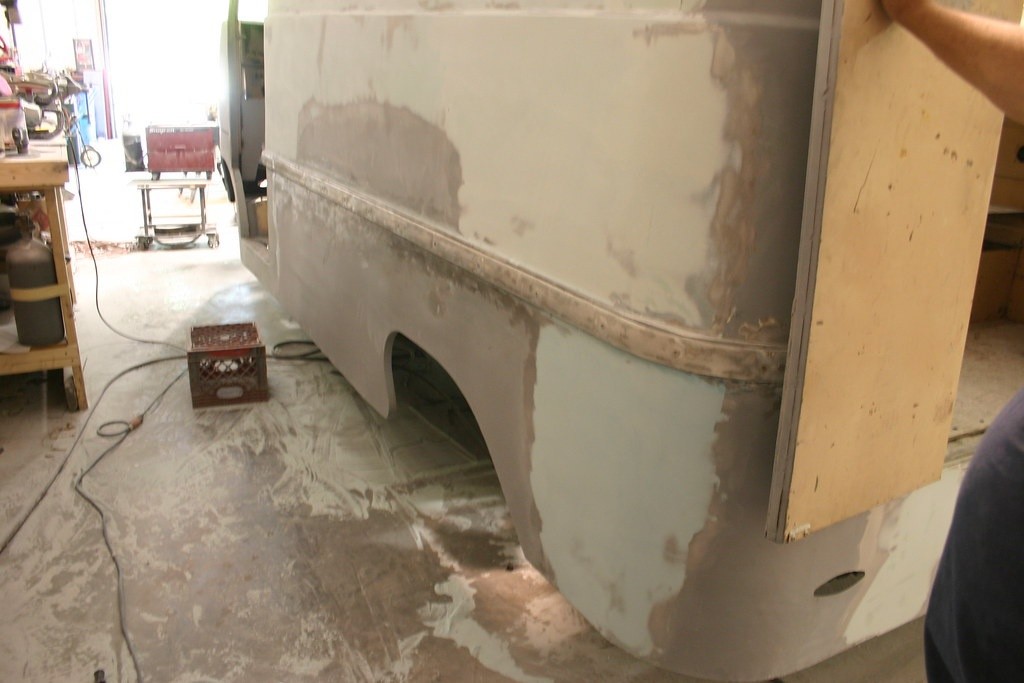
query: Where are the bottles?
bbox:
[0,101,29,155]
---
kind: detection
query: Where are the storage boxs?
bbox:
[187,322,269,408]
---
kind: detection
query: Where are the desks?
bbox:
[134,179,220,250]
[0,122,88,413]
